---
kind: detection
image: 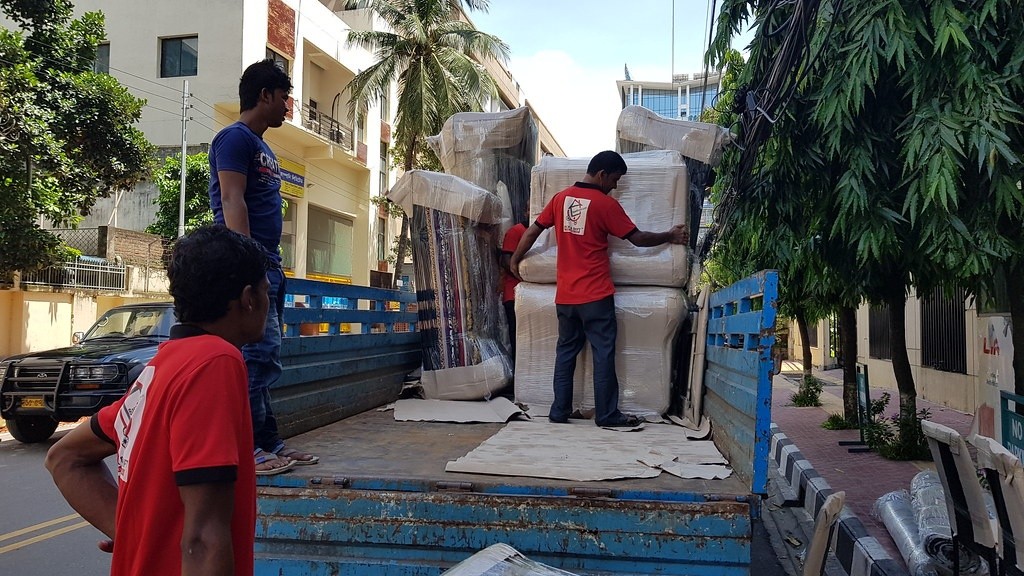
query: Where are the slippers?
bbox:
[277,448,320,464]
[254,453,297,475]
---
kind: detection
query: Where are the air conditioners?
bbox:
[307,120,323,135]
[329,128,346,143]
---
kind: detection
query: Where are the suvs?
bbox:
[0,301,182,443]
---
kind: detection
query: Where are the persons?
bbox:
[503,197,530,400]
[509,151,690,428]
[44,223,270,576]
[207,57,320,474]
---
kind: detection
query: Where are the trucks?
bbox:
[254,268,779,576]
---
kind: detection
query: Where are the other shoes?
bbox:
[600,412,646,431]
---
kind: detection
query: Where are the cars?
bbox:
[35,266,75,286]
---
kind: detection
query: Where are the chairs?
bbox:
[140,325,159,335]
